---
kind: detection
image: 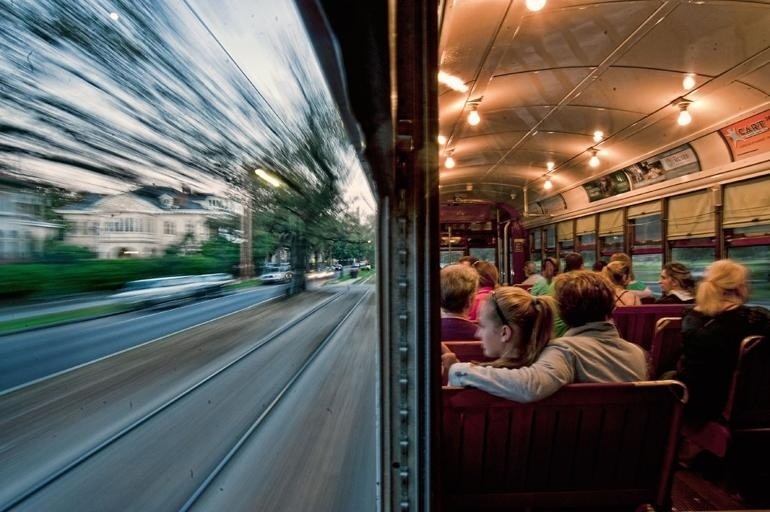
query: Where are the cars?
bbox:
[258,263,293,283]
[111,271,233,306]
[689,266,708,279]
[350,260,368,268]
[332,264,343,271]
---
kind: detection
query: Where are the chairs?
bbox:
[442,379,689,511]
[442,283,767,506]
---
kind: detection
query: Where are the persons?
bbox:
[465,286,561,372]
[440,265,480,342]
[530,257,569,337]
[441,268,651,403]
[566,252,585,271]
[515,260,540,285]
[460,256,479,265]
[469,260,499,320]
[592,261,607,272]
[603,260,641,307]
[660,256,770,469]
[656,262,697,304]
[611,252,646,291]
